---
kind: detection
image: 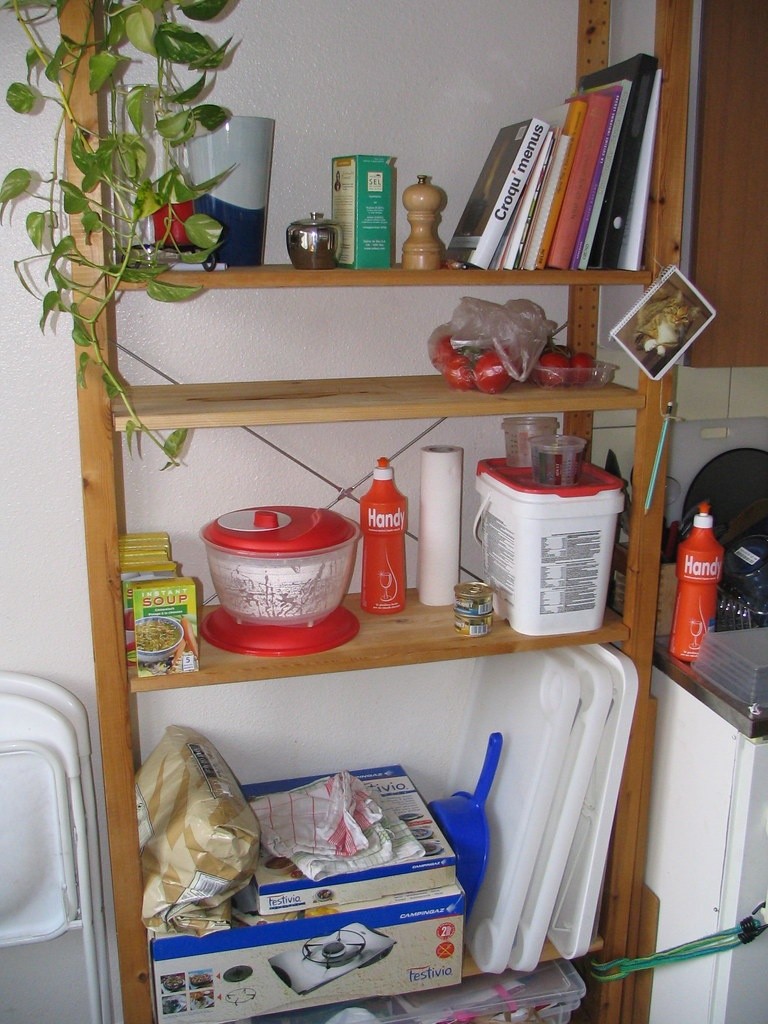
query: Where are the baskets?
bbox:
[714,585,768,632]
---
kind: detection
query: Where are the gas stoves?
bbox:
[268,922,396,995]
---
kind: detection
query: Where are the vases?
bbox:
[185,116,275,268]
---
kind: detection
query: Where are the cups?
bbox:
[286,211,343,269]
[189,116,276,268]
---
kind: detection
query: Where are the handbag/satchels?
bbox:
[425,295,557,396]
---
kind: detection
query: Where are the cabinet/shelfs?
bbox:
[53,0,694,1024]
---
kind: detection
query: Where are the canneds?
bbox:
[452,581,493,637]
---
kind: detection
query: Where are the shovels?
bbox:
[430,732,505,921]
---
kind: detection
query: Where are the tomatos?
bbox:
[434,333,595,393]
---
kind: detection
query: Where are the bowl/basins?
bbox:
[123,608,135,645]
[136,616,184,660]
[503,416,559,468]
[530,435,585,486]
[200,508,363,628]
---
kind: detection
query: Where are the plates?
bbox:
[314,889,333,903]
[409,826,433,839]
[191,975,212,984]
[418,840,444,855]
[190,996,214,1011]
[163,976,183,991]
[262,854,294,871]
[163,999,186,1014]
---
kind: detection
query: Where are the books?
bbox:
[444,52,662,271]
[610,264,716,381]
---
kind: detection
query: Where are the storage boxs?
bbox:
[332,154,391,270]
[612,541,680,635]
[473,456,623,635]
[118,532,201,678]
[146,765,587,1024]
